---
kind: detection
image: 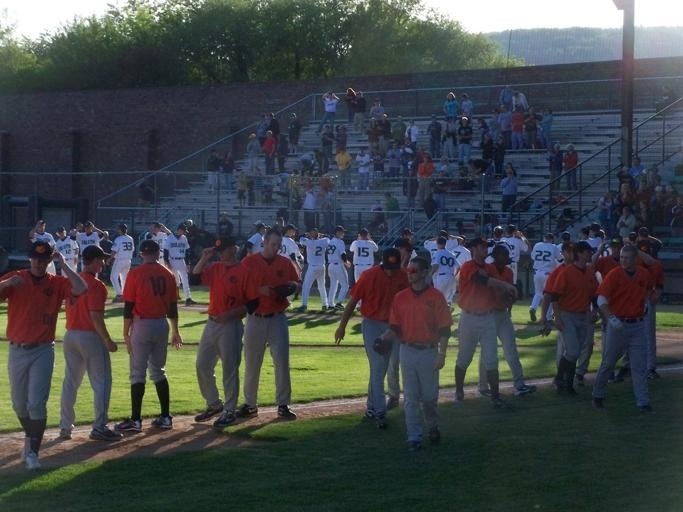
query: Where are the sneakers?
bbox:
[366,399,399,430]
[639,406,655,416]
[196,403,258,427]
[89,418,142,441]
[186,299,195,305]
[152,415,173,429]
[60,428,72,438]
[407,441,421,452]
[23,443,41,470]
[479,388,512,409]
[591,397,604,412]
[513,384,537,396]
[529,309,537,321]
[454,391,465,407]
[334,304,344,310]
[429,426,440,441]
[551,374,584,396]
[277,407,296,419]
[607,368,660,384]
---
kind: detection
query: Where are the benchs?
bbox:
[115,107,683,232]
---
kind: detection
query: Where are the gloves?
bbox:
[432,352,445,369]
[538,322,551,337]
[609,315,624,330]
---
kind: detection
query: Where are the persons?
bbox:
[385,237,413,412]
[135,177,158,224]
[53,243,125,446]
[332,245,408,434]
[30,219,195,304]
[1,238,89,474]
[655,84,679,115]
[475,240,538,401]
[453,237,518,414]
[400,223,663,326]
[243,222,380,312]
[538,238,666,416]
[598,158,681,242]
[206,87,577,229]
[111,239,184,432]
[238,228,305,423]
[373,254,453,455]
[185,232,262,430]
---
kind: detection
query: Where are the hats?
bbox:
[287,224,298,231]
[335,226,347,232]
[545,223,649,251]
[179,222,189,234]
[83,245,111,258]
[140,240,160,252]
[27,241,52,259]
[469,238,494,247]
[383,247,400,270]
[213,237,232,251]
[403,228,414,235]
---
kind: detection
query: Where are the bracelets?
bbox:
[103,337,110,344]
[438,353,448,358]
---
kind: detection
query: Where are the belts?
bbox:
[626,319,642,324]
[18,343,37,350]
[254,313,272,318]
[407,342,430,350]
